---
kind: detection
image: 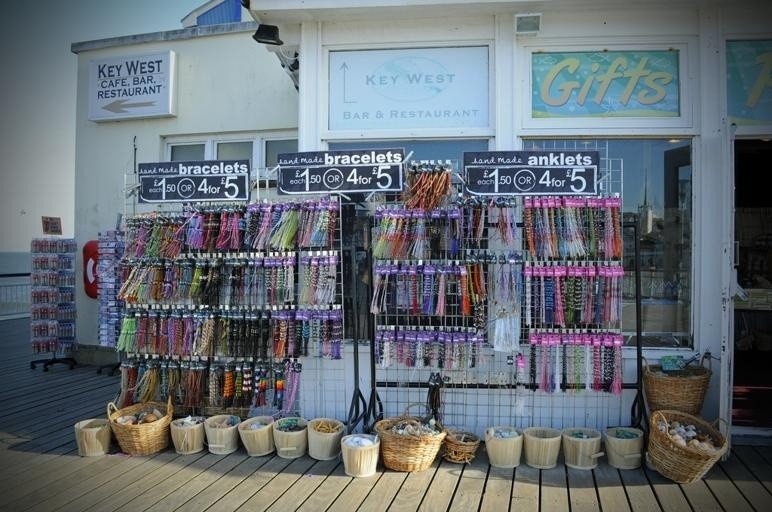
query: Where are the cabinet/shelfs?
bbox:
[117,171,348,424]
[28,237,79,372]
[371,155,626,434]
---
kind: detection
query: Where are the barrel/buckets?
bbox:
[169,415,383,478]
[481,424,642,472]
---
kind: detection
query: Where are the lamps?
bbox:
[513,12,543,36]
[252,24,285,46]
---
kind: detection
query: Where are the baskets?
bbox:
[559,427,601,472]
[648,409,729,486]
[202,414,241,455]
[306,415,345,461]
[520,424,562,470]
[482,424,522,469]
[107,395,175,456]
[170,414,206,455]
[341,431,380,477]
[238,413,275,457]
[370,403,448,472]
[603,425,644,471]
[273,416,309,458]
[441,423,479,463]
[639,350,713,416]
